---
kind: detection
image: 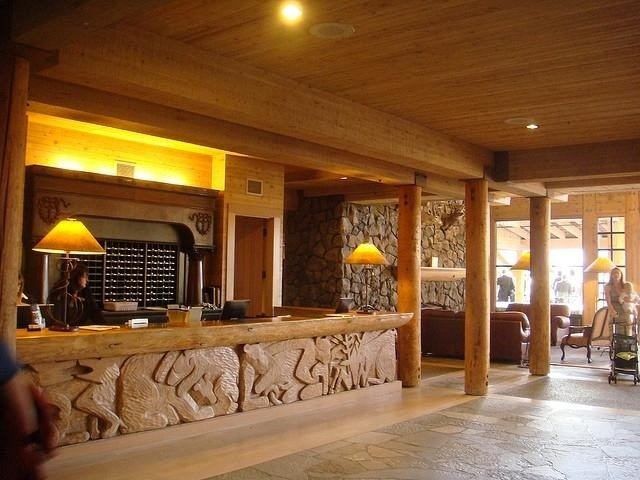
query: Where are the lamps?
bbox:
[510,252,530,270]
[341,243,388,314]
[583,257,618,274]
[32,216,107,333]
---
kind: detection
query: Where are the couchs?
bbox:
[422,302,571,367]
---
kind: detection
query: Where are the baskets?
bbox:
[103,301,138,312]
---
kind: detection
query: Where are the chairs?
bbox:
[560,306,609,363]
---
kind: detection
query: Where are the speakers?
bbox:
[494,151,509,181]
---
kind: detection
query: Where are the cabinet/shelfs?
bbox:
[72,238,179,312]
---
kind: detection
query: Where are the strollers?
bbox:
[608,311,640,384]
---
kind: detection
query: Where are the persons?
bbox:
[618,283,639,315]
[551,268,577,306]
[496,269,514,302]
[47,263,104,328]
[605,267,635,338]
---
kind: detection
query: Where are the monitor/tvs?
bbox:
[335,298,353,313]
[220,300,250,321]
